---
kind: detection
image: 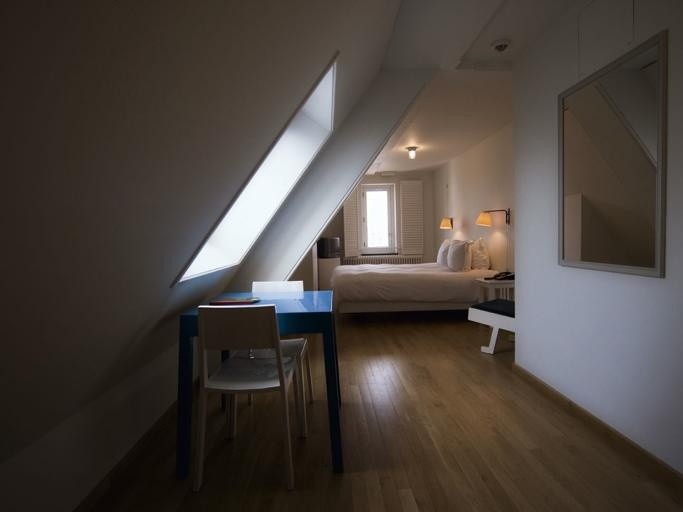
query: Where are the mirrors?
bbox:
[554,27,669,281]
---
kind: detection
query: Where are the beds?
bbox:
[330,261,500,316]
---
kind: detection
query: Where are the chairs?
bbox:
[230,279,315,440]
[191,299,307,490]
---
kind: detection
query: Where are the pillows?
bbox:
[469,236,493,270]
[436,237,460,268]
[446,237,472,272]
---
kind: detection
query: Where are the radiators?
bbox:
[342,254,423,265]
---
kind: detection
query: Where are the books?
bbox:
[209,297,260,305]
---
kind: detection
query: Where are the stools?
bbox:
[465,297,514,356]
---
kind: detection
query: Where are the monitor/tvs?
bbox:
[318,237,340,258]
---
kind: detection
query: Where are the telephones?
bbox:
[494,272,515,280]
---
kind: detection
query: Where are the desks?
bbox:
[173,287,348,479]
[472,276,515,326]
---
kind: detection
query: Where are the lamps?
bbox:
[438,216,453,231]
[404,146,418,160]
[474,207,511,228]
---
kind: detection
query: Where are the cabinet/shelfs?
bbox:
[317,256,341,291]
